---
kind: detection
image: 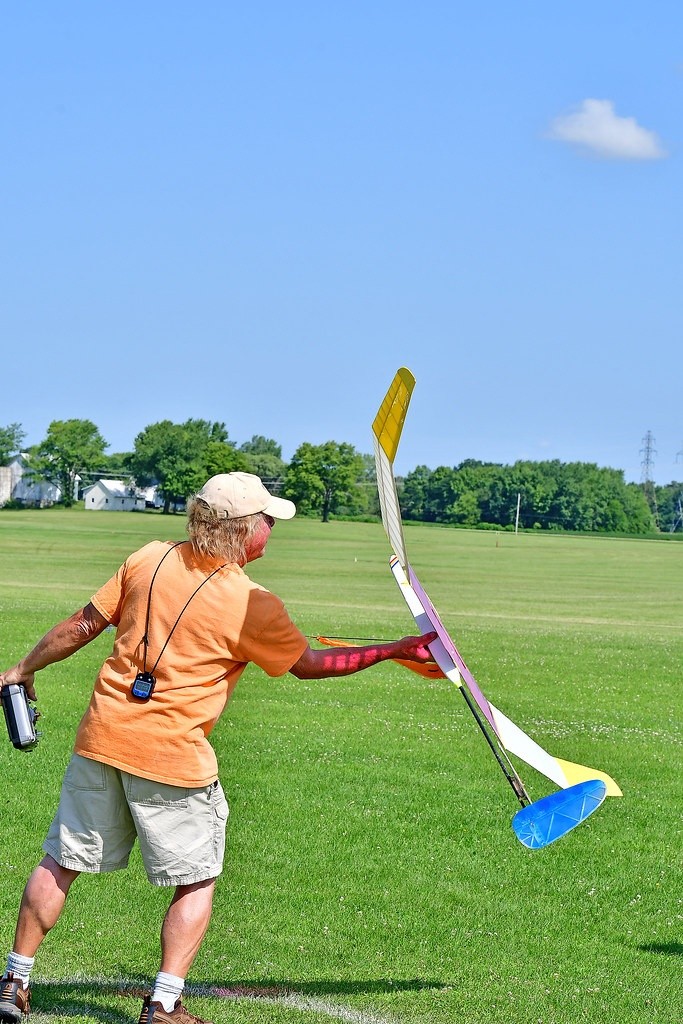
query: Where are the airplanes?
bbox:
[296,366,623,850]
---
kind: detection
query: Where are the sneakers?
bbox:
[138,995,214,1024]
[0,972,32,1024]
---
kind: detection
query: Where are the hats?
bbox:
[194,472,296,520]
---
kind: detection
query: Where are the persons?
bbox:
[0,472,438,1024]
